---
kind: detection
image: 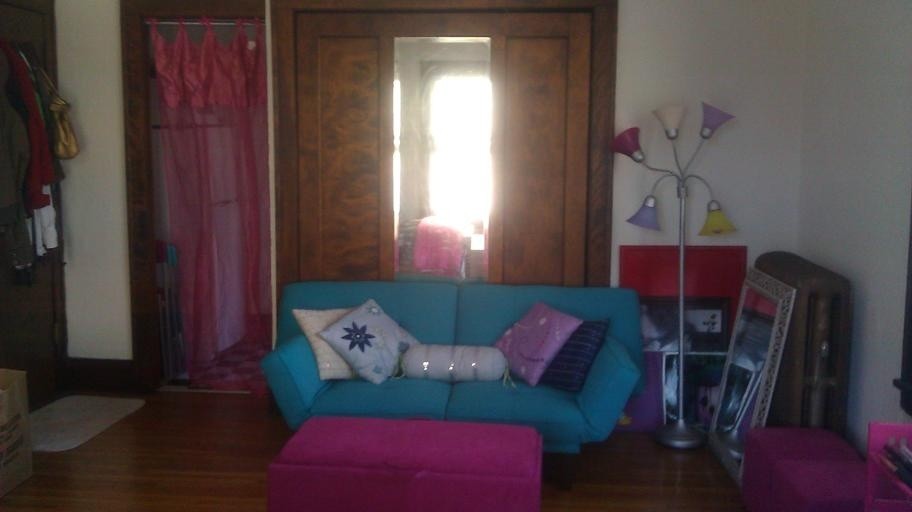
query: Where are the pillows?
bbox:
[290,306,362,380]
[539,317,611,392]
[493,299,586,387]
[317,296,421,386]
[401,342,508,382]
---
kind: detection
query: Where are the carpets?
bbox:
[29,394,146,453]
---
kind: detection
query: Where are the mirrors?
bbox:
[378,25,512,285]
[708,265,799,486]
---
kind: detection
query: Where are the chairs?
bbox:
[741,427,868,512]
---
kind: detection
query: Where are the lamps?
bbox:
[605,100,739,451]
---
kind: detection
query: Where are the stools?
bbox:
[266,415,545,512]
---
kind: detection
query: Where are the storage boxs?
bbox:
[0,367,34,499]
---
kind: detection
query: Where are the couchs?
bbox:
[258,278,645,456]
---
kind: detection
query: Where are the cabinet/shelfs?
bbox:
[618,243,748,434]
[866,421,912,512]
